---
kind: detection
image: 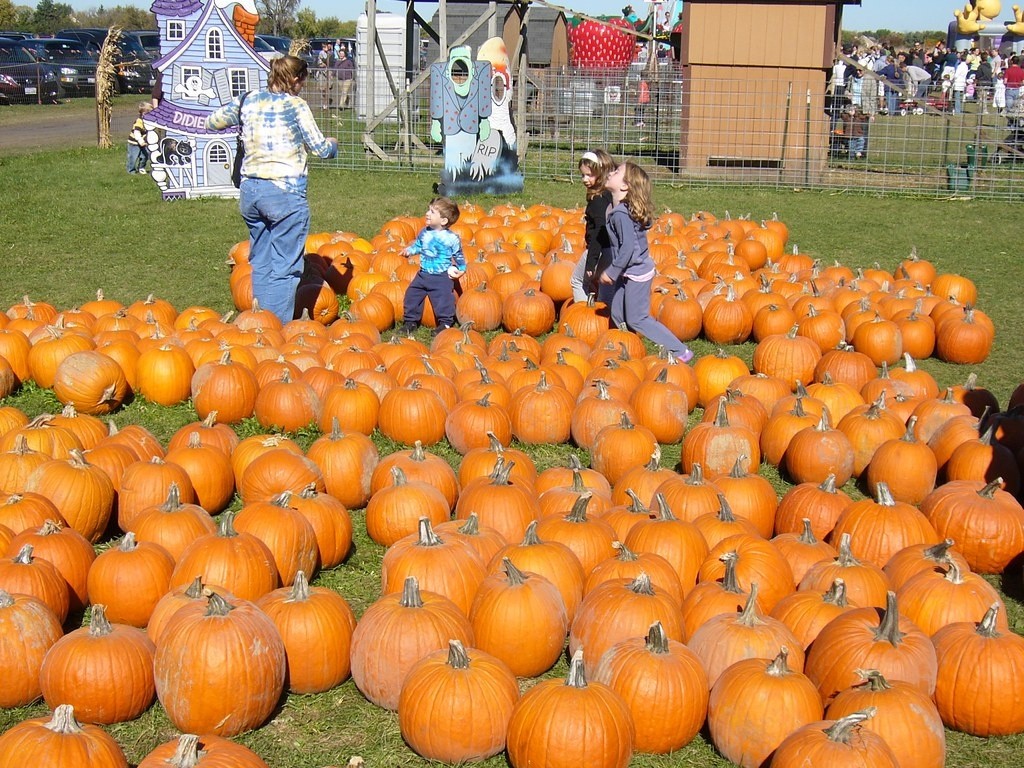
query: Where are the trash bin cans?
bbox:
[966,144,988,169]
[946,164,973,191]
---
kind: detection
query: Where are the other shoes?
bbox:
[138,168,148,175]
[430,323,451,337]
[675,348,697,365]
[400,322,417,335]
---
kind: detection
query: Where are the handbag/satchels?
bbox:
[231,135,247,188]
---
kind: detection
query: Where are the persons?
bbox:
[152,72,165,109]
[633,77,651,127]
[127,102,154,175]
[828,38,1024,156]
[315,39,354,109]
[204,55,338,326]
[570,148,620,303]
[398,197,467,337]
[656,9,684,64]
[840,104,874,158]
[599,161,695,364]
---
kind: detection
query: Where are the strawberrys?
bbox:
[567,13,638,85]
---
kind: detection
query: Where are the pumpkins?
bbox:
[0,290,1024,768]
[226,201,996,367]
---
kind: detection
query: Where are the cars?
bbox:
[19,39,100,98]
[0,37,58,105]
[307,38,357,61]
[58,28,159,95]
[255,34,314,74]
[128,30,161,60]
[0,31,33,40]
[253,36,284,62]
